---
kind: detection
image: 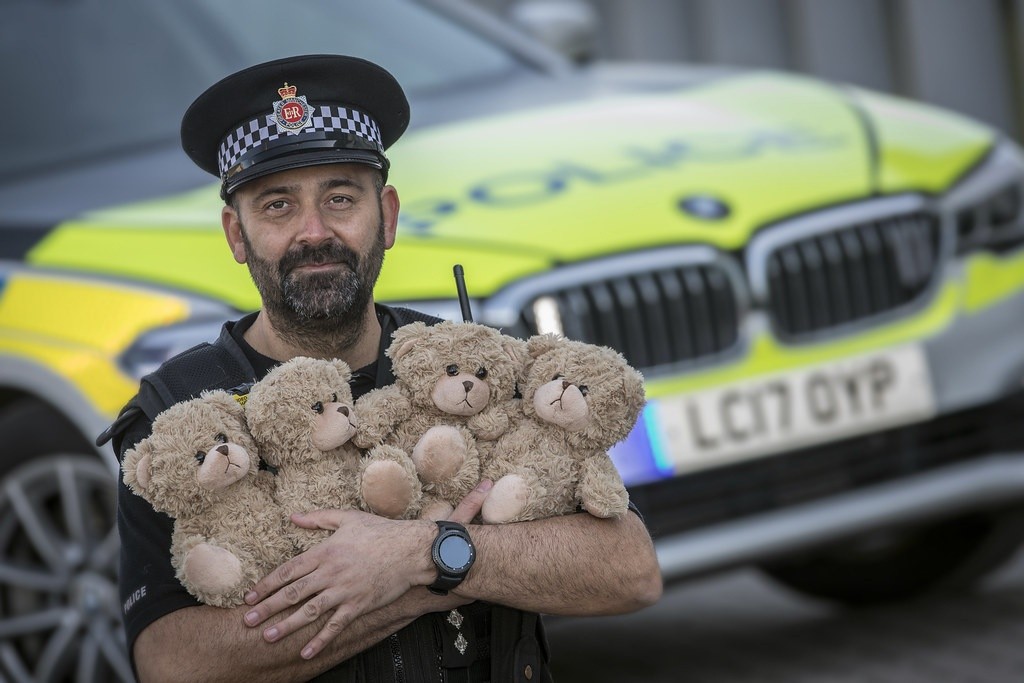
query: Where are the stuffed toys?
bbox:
[120,389,298,608]
[352,320,526,520]
[468,332,646,523]
[245,356,365,553]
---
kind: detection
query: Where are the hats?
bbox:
[181,54,411,202]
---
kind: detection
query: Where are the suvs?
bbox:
[0,0,1023,682]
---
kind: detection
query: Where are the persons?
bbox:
[96,54,663,683]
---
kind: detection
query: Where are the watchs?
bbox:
[426,521,476,596]
